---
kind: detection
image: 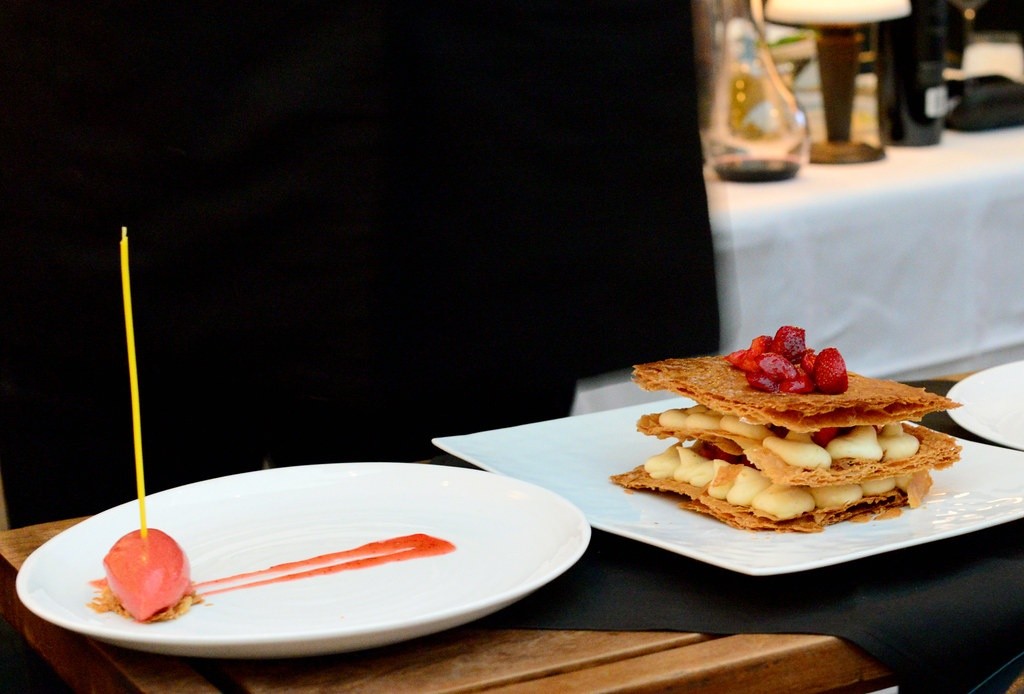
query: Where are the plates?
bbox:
[15,461,593,661]
[945,360,1024,452]
[431,397,1024,577]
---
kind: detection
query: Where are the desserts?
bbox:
[612,327,964,535]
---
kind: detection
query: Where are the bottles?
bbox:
[696,0,809,180]
[872,0,949,142]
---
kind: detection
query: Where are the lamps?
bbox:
[764,1,914,164]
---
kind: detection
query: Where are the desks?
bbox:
[697,25,1024,379]
